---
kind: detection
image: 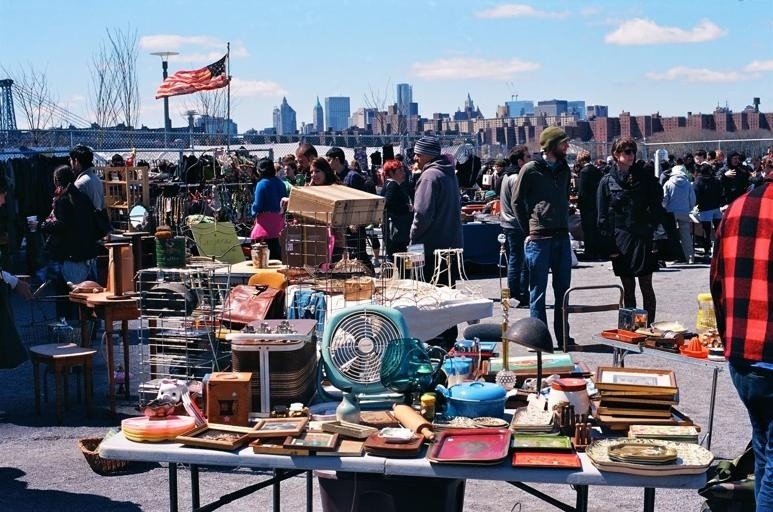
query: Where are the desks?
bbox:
[591,333,730,452]
[67,287,156,422]
[283,277,493,375]
[460,212,511,273]
[97,408,708,512]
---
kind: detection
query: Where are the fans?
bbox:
[321,305,413,420]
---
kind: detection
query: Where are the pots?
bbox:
[434,380,518,420]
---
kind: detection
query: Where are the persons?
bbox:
[139,159,150,167]
[69,146,105,328]
[576,150,604,261]
[408,136,464,351]
[309,158,343,185]
[456,144,481,188]
[481,168,496,191]
[695,164,722,255]
[496,159,509,196]
[717,152,756,206]
[251,159,289,261]
[661,150,725,172]
[739,150,773,182]
[350,160,380,261]
[395,155,410,195]
[512,127,580,347]
[710,168,773,511]
[326,147,376,279]
[603,155,616,176]
[500,144,531,304]
[0,174,32,369]
[275,155,297,187]
[597,136,664,328]
[661,165,696,264]
[295,145,318,184]
[113,154,128,166]
[30,165,113,321]
[594,155,606,174]
[382,161,416,279]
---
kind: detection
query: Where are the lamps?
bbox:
[500,316,555,398]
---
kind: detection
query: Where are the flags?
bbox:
[157,53,230,98]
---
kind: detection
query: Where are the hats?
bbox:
[414,135,441,156]
[539,126,569,152]
[480,154,493,164]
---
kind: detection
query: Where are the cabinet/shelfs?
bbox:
[90,166,151,243]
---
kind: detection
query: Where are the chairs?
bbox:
[462,298,510,343]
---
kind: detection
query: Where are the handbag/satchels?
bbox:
[83,191,114,240]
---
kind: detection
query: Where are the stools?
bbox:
[29,341,98,419]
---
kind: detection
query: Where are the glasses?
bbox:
[730,157,741,161]
[614,148,638,156]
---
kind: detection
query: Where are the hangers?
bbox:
[224,182,227,192]
[156,182,254,187]
[221,182,224,191]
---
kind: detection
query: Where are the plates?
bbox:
[509,432,573,451]
[230,319,318,408]
[649,320,687,331]
[585,438,716,475]
[508,407,561,436]
[376,426,415,442]
[121,415,195,444]
[607,442,677,465]
[425,429,513,467]
[361,430,425,458]
[510,451,583,469]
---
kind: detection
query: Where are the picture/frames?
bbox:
[589,366,701,435]
[321,419,378,439]
[282,429,340,452]
[176,424,254,451]
[247,417,308,437]
